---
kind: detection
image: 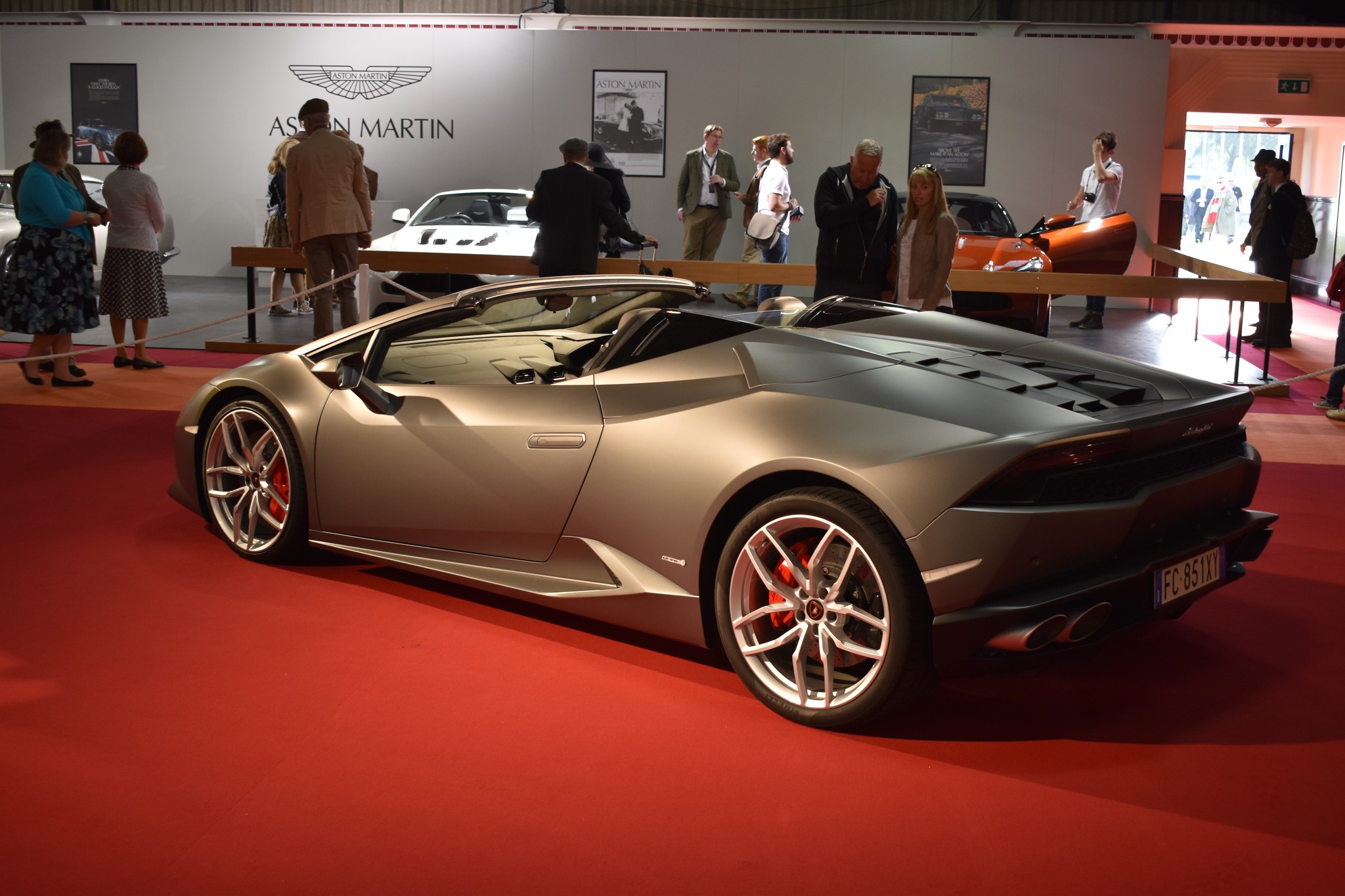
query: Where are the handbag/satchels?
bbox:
[745,212,781,250]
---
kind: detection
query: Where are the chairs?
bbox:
[460,199,508,224]
[581,307,662,375]
[952,215,972,234]
[753,296,807,328]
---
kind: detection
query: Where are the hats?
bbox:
[1251,149,1275,161]
[30,120,74,148]
[559,138,589,154]
[585,142,614,169]
[298,98,329,119]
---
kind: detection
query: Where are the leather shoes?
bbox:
[748,299,757,306]
[723,292,745,308]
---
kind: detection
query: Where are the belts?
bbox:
[698,204,721,210]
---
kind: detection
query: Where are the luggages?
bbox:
[638,241,673,277]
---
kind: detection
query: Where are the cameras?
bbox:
[1083,193,1096,203]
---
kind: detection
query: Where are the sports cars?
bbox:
[888,191,1137,337]
[166,273,1282,731]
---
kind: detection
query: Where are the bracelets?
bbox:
[84,212,88,223]
[787,202,793,210]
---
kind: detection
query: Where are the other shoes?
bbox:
[308,298,338,309]
[1079,315,1104,330]
[1070,317,1092,326]
[17,356,165,387]
[1242,331,1262,341]
[1284,337,1292,348]
[702,295,715,302]
[1253,339,1286,348]
[332,297,340,304]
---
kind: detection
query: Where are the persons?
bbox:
[1067,131,1123,329]
[618,100,644,151]
[1313,253,1345,422]
[677,125,740,301]
[1240,149,1302,348]
[758,133,802,307]
[263,139,313,316]
[813,139,898,303]
[0,119,112,387]
[288,130,378,307]
[1181,177,1243,244]
[892,164,959,316]
[98,132,170,369]
[526,138,658,279]
[722,135,771,309]
[585,141,631,258]
[286,99,373,340]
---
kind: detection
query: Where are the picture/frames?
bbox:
[908,74,991,188]
[591,67,668,178]
[69,62,139,166]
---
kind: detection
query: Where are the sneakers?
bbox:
[268,304,298,317]
[296,301,313,315]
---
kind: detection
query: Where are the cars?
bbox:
[0,168,182,288]
[77,118,124,150]
[594,112,664,147]
[914,93,984,134]
[353,188,540,319]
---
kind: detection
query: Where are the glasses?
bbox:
[1229,180,1234,182]
[914,163,939,178]
[1217,182,1224,184]
[708,134,724,139]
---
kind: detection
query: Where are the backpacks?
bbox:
[1283,185,1318,261]
